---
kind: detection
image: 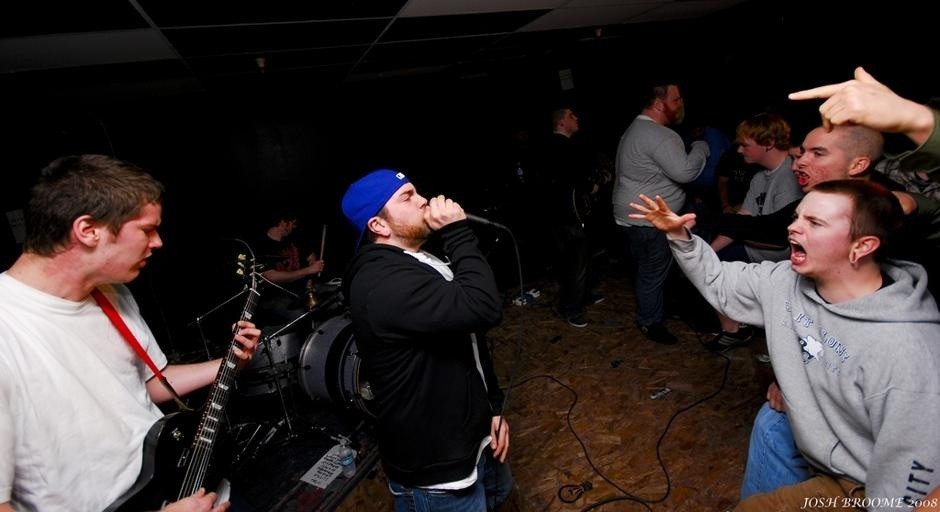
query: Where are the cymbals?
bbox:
[286,285,338,311]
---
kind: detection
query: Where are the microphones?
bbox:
[465,213,506,230]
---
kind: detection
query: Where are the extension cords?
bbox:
[511,287,541,307]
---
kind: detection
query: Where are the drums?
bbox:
[297,315,378,417]
[233,326,300,397]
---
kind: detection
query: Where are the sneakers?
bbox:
[556,290,605,329]
[636,319,678,345]
[704,325,752,354]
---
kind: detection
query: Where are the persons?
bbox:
[506,64,940,512]
[243,209,326,338]
[339,167,513,512]
[1,151,263,512]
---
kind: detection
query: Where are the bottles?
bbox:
[512,162,527,184]
[305,289,317,311]
[340,438,356,480]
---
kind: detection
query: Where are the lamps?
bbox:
[559,67,574,92]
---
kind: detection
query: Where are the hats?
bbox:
[340,169,409,253]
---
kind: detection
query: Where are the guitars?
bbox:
[103,253,265,512]
